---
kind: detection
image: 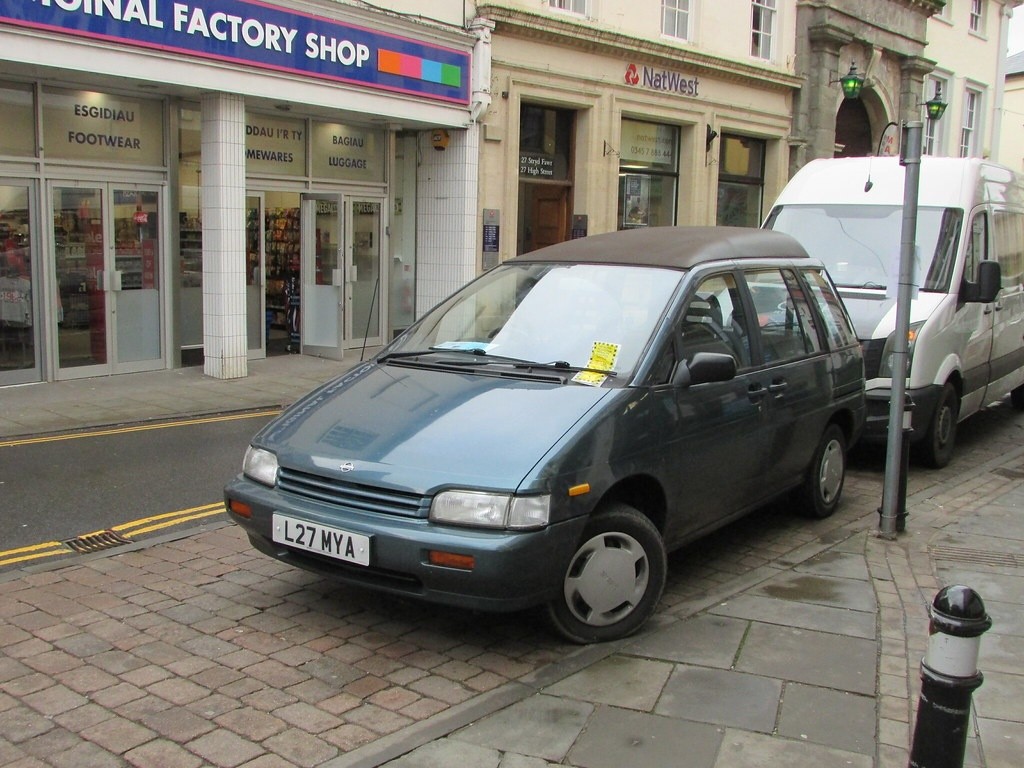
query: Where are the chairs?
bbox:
[682,292,733,358]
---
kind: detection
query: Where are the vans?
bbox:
[703,154,1023,468]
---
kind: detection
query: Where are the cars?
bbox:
[225,221,868,647]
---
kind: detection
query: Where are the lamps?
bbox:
[915,87,949,120]
[828,58,865,100]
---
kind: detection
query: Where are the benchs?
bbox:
[722,327,802,366]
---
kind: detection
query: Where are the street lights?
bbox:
[839,59,950,541]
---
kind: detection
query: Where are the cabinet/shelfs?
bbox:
[179,228,202,251]
[65,254,142,288]
